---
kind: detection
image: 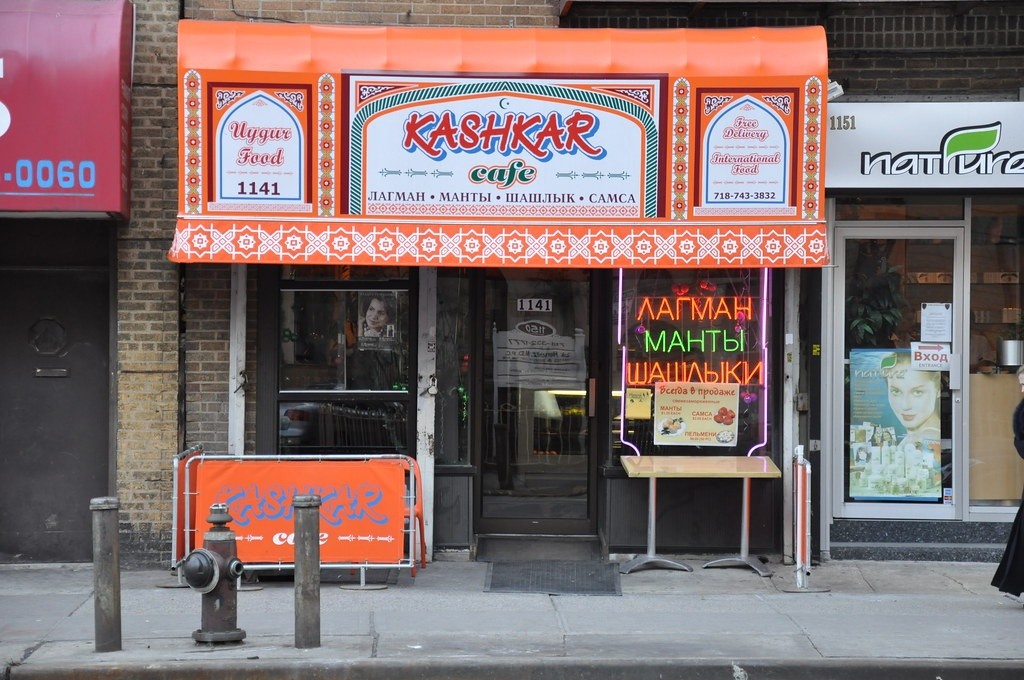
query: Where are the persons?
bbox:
[365,292,396,338]
[991,365,1024,597]
[851,351,941,465]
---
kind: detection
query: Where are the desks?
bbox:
[620,455,782,577]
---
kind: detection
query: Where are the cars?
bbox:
[279,383,405,455]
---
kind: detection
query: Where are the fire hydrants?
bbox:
[171,502,246,645]
